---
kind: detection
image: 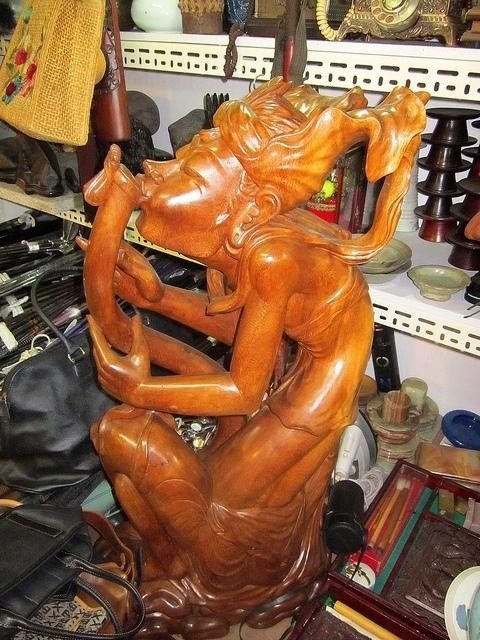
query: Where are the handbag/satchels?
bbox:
[1,0,107,146]
[1,265,219,494]
[0,502,147,639]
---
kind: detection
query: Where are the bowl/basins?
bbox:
[408,264,470,301]
[352,234,411,274]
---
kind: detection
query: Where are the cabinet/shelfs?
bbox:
[0,29,480,358]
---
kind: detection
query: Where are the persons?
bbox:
[73,73,433,640]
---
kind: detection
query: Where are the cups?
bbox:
[324,481,364,554]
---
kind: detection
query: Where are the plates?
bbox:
[444,567,480,640]
[466,582,480,640]
